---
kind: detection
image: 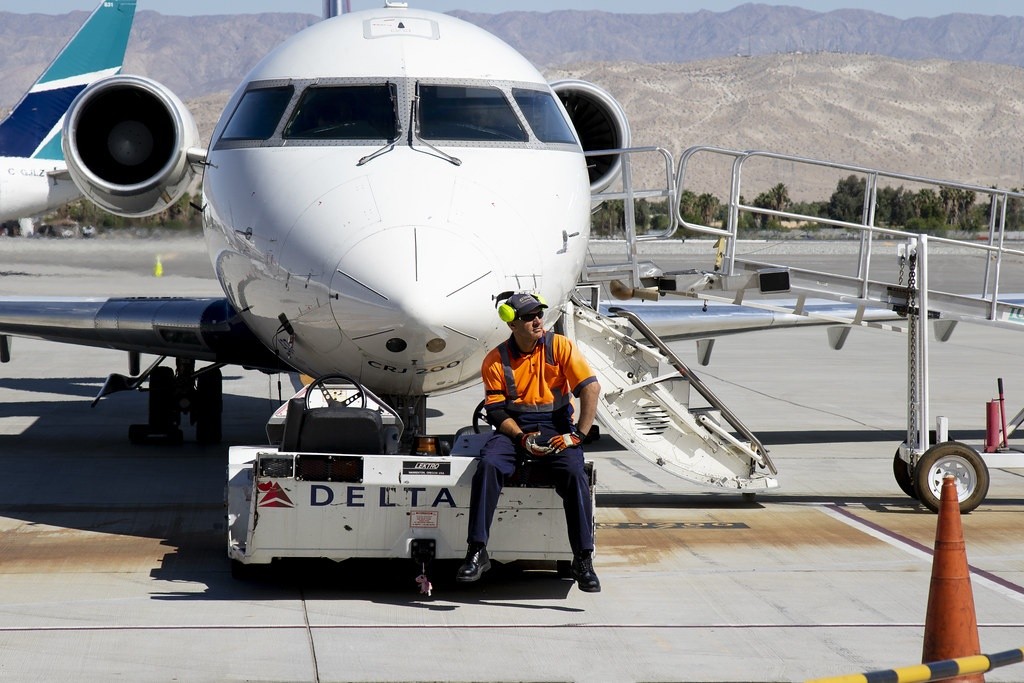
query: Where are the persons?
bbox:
[457,294,601,593]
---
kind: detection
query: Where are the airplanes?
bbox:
[0,0,137,225]
[0,1,1024,604]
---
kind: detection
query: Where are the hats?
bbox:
[505,294,548,319]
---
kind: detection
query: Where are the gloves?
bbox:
[515,432,551,455]
[547,430,586,453]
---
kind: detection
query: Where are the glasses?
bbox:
[516,310,543,322]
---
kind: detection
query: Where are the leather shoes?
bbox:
[457,543,491,582]
[569,550,601,592]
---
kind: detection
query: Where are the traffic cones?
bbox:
[920,476,987,683]
